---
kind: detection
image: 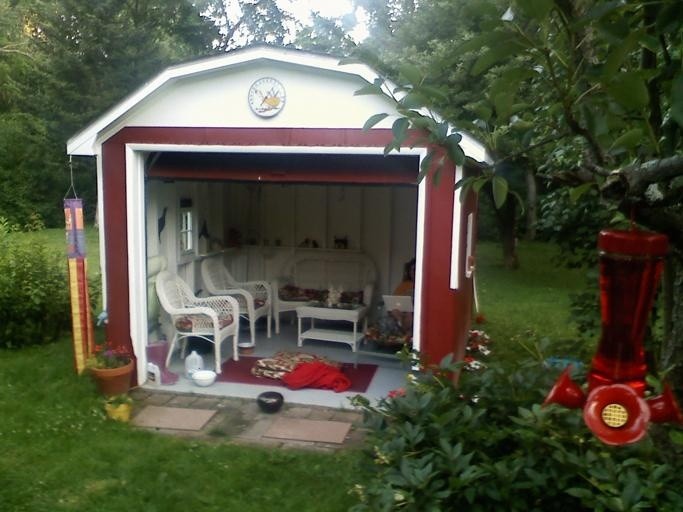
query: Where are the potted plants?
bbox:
[97,392,143,423]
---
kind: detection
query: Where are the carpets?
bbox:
[213,357,379,393]
[264,416,351,443]
[131,406,219,432]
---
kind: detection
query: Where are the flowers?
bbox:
[84,341,130,369]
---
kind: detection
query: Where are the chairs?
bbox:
[200,257,272,343]
[156,271,240,375]
[363,263,413,347]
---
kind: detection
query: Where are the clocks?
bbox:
[248,77,286,118]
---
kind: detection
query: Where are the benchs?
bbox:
[265,254,376,344]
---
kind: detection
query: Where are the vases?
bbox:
[90,358,134,395]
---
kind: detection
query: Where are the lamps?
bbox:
[541,210,683,446]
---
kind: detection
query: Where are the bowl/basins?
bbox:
[238,343,256,355]
[192,370,217,387]
[256,391,284,414]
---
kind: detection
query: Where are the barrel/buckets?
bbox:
[184,350,204,381]
[547,359,587,386]
